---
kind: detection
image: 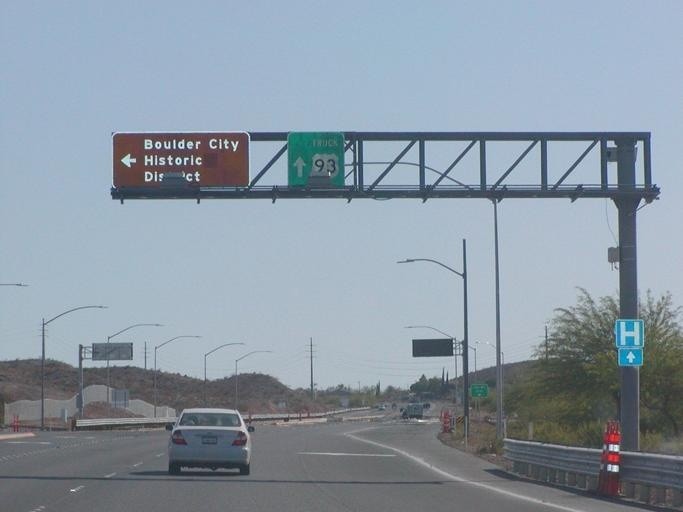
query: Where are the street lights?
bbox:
[204,341,245,410]
[0,281,25,292]
[38,304,117,435]
[484,340,505,399]
[396,235,472,445]
[405,325,479,417]
[153,334,203,420]
[105,320,163,417]
[234,348,276,415]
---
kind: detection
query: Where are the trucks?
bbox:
[400,402,424,420]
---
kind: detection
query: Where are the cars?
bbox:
[165,405,256,476]
[379,404,385,411]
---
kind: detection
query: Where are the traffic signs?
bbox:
[112,132,250,189]
[288,131,345,192]
[470,383,488,398]
